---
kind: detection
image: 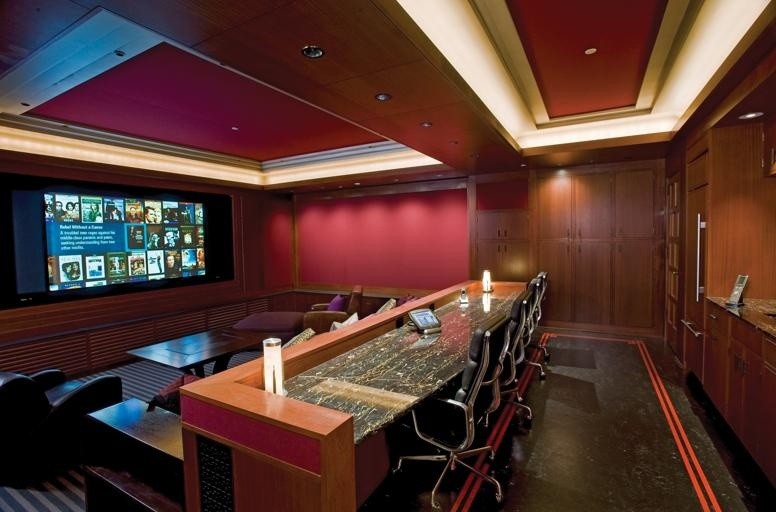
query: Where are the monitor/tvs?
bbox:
[0,171,234,311]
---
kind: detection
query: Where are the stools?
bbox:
[233,312,304,352]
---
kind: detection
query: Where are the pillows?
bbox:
[327,293,344,311]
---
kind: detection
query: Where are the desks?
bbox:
[125,327,266,378]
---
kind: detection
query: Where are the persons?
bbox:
[197,247,206,271]
[55,201,80,220]
[89,202,100,223]
[145,205,163,224]
[126,206,140,222]
[104,202,122,222]
[62,261,79,282]
[165,253,180,277]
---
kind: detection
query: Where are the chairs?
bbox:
[388,272,550,512]
[304,286,363,335]
[0,369,122,486]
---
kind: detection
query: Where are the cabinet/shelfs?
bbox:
[475,209,530,282]
[681,152,708,386]
[703,300,776,492]
[85,398,185,512]
[536,169,655,329]
